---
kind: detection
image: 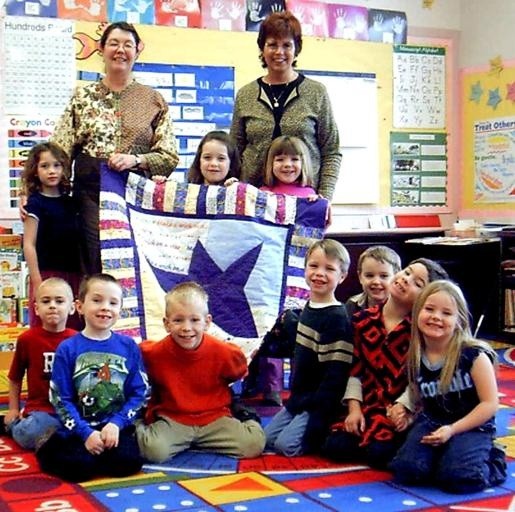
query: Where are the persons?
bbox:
[321,253,450,466]
[21,18,181,272]
[346,244,403,317]
[150,133,244,187]
[22,139,86,334]
[3,276,82,451]
[132,281,270,466]
[34,272,150,482]
[233,7,341,230]
[382,277,508,496]
[262,234,356,462]
[258,133,326,203]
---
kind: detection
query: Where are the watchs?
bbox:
[131,153,142,170]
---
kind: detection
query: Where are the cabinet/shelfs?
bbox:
[321,226,515,346]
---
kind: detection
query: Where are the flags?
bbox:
[91,162,326,401]
